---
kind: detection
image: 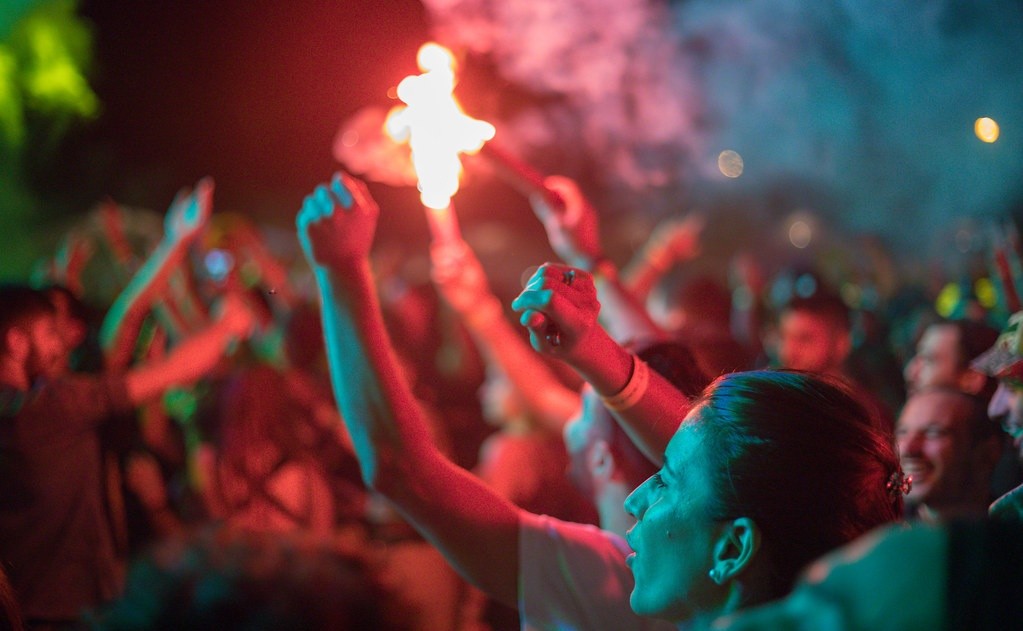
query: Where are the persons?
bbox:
[0,173,1022,630]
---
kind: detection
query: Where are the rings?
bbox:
[567,270,575,287]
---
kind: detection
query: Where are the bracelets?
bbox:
[599,348,649,412]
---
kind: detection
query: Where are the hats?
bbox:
[970,311,1023,379]
